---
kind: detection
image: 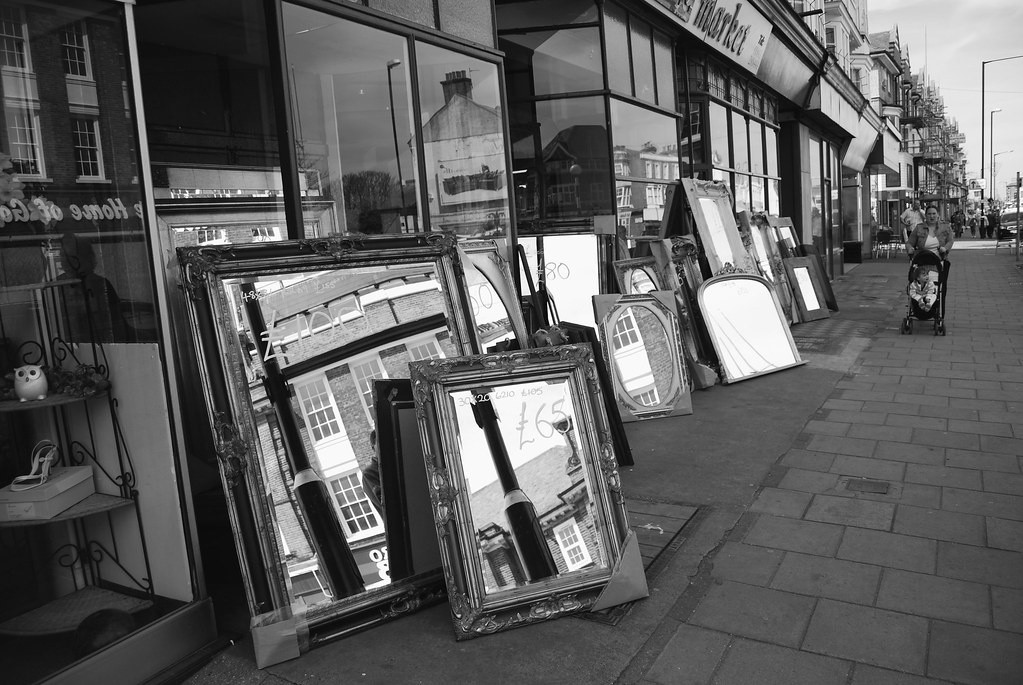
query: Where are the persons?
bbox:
[900,198,926,262]
[910,266,937,311]
[951,210,996,238]
[905,206,953,270]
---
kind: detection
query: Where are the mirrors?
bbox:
[151,176,838,671]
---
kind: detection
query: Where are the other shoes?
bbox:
[925,303,931,312]
[919,302,925,310]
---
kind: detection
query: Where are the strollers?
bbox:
[900,246,950,337]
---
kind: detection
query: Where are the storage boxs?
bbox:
[0,465,96,522]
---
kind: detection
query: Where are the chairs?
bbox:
[876,230,893,260]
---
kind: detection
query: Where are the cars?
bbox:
[995,210,1023,243]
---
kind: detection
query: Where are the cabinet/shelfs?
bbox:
[0,277,153,638]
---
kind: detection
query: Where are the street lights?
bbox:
[989,108,1003,212]
[570,163,584,218]
[993,148,1016,205]
[386,58,410,233]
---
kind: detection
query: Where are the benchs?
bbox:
[994,236,1023,255]
